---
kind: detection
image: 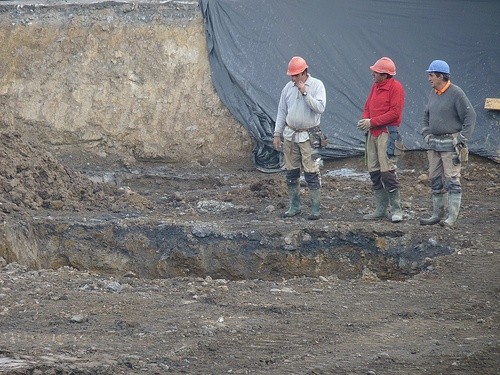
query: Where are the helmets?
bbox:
[370,57,396,75]
[287,56,308,75]
[426,60,450,74]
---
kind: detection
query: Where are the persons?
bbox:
[420,60,476,227]
[273,56,326,220]
[357,57,405,221]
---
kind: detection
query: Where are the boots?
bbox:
[363,188,388,219]
[308,189,320,220]
[444,192,462,227]
[387,189,403,222]
[284,183,300,217]
[420,193,445,225]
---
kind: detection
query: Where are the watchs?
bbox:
[302,91,307,96]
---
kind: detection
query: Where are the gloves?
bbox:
[357,118,371,132]
